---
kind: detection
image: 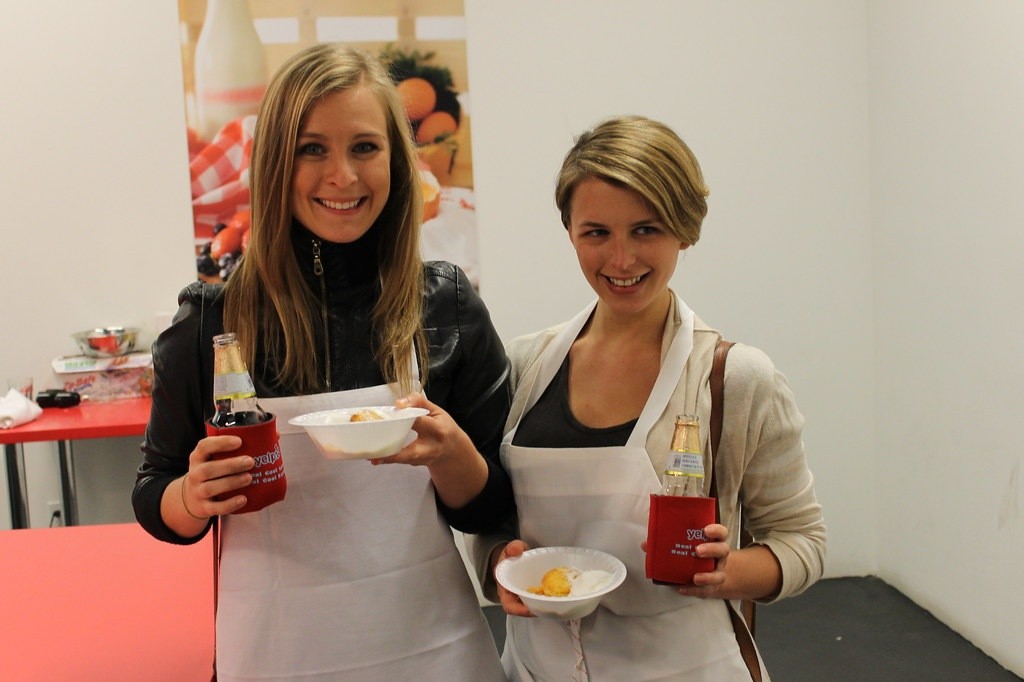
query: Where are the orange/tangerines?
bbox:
[396,77,456,146]
[210,210,252,259]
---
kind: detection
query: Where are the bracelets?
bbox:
[181,474,206,520]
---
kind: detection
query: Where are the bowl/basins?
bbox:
[70,327,141,359]
[288,405,430,461]
[496,547,627,620]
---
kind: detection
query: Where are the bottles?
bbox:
[663,414,707,497]
[212,333,268,428]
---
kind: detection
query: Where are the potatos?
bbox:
[540,567,576,596]
[350,410,385,423]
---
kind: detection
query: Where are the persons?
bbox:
[464,115,827,681]
[132,42,511,682]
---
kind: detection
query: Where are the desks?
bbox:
[0,512,221,682]
[0,390,156,530]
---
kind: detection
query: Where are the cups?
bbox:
[646,493,716,586]
[206,412,287,515]
